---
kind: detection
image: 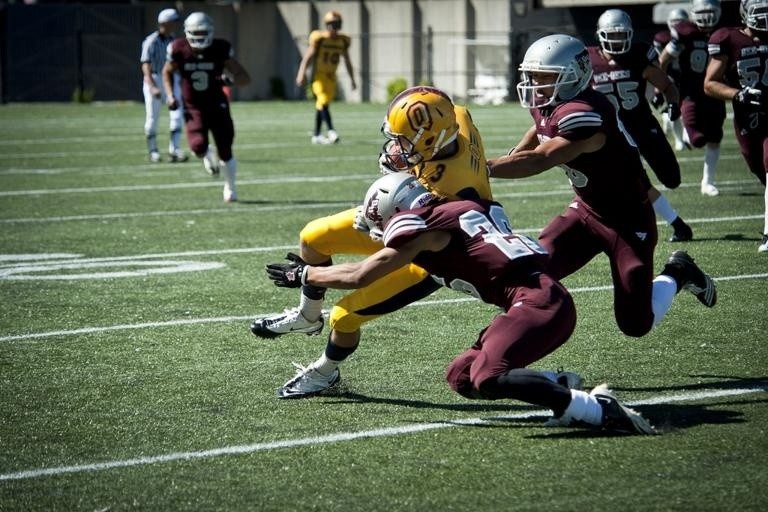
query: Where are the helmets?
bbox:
[667,0,768,32]
[516,8,634,109]
[362,173,429,242]
[184,12,215,50]
[380,85,458,172]
[324,11,342,28]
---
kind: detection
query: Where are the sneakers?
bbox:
[251,306,324,339]
[701,179,720,197]
[548,366,660,437]
[311,130,340,145]
[668,225,693,242]
[665,250,717,308]
[276,360,340,399]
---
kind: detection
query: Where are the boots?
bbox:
[146,128,237,203]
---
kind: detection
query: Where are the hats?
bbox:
[157,8,180,24]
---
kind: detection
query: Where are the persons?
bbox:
[162,12,251,202]
[489,0,767,335]
[139,8,190,164]
[266,172,658,435]
[249,83,494,399]
[294,10,357,145]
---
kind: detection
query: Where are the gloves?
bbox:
[736,84,763,106]
[650,92,681,121]
[265,252,311,288]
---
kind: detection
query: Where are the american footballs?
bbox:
[388,145,407,167]
